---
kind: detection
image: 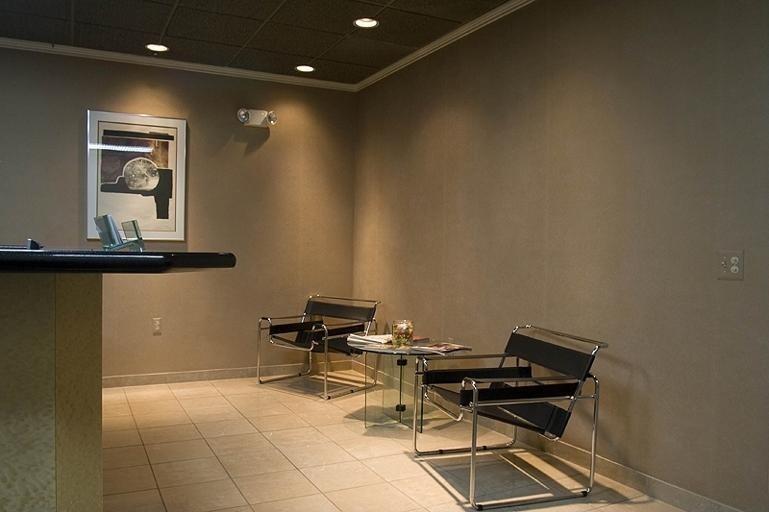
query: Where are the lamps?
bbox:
[236,105,280,131]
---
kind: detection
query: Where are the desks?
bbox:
[1,242,239,512]
[347,330,474,433]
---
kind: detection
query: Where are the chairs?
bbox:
[410,322,610,511]
[254,292,382,402]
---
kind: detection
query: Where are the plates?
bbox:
[121,156,160,193]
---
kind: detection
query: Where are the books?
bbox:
[413,341,472,356]
[412,334,429,342]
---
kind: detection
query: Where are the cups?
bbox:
[391,319,414,352]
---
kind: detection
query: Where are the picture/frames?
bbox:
[84,107,189,244]
